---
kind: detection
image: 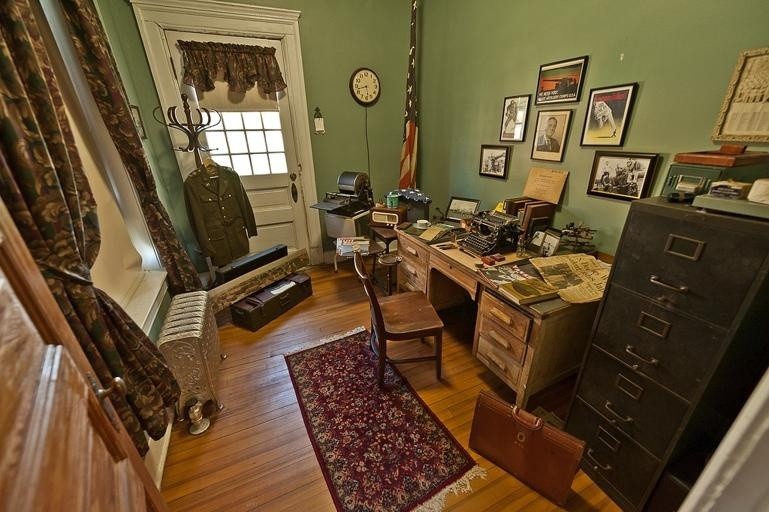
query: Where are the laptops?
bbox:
[437,196,481,229]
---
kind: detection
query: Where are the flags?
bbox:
[398,1,425,195]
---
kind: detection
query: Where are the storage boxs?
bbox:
[228,270,314,334]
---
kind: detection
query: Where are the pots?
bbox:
[384,192,400,209]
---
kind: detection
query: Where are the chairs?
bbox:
[351,250,444,390]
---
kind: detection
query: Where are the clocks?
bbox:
[349,68,382,108]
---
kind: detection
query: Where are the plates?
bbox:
[413,223,426,230]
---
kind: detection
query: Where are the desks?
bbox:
[394,219,615,412]
[323,194,430,295]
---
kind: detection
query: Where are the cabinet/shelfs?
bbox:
[564,194,769,511]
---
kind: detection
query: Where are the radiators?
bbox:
[156,290,231,435]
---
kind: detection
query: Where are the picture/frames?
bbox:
[530,110,574,162]
[445,197,481,223]
[130,102,149,140]
[480,144,512,179]
[580,80,638,148]
[533,55,590,105]
[709,47,769,144]
[585,149,662,202]
[498,94,532,142]
[537,231,560,259]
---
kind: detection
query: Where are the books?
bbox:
[477,263,558,306]
[335,236,370,257]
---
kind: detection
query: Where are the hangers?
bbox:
[201,145,219,180]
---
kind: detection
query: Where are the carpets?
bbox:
[283,326,490,511]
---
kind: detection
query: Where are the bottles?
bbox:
[516,237,526,257]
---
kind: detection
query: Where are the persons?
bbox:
[537,116,561,153]
[503,100,518,134]
[599,157,644,196]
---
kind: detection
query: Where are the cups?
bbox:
[416,219,431,228]
